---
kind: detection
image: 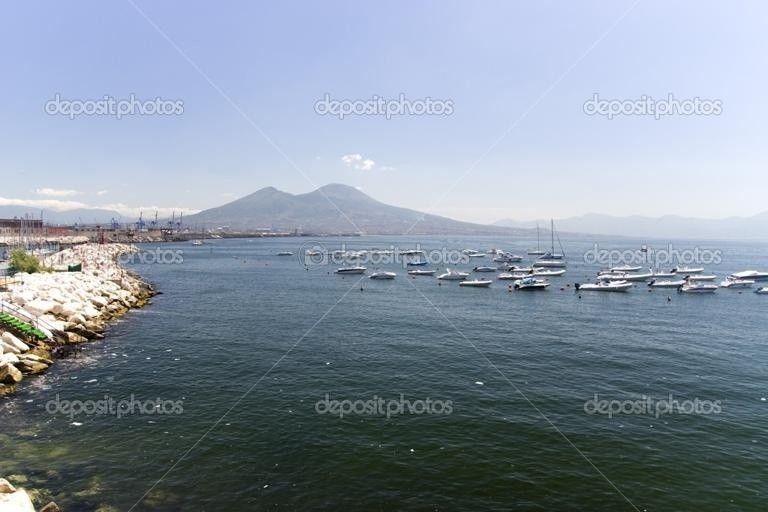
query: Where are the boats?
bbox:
[191,240,202,246]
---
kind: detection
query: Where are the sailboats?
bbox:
[4,208,61,258]
